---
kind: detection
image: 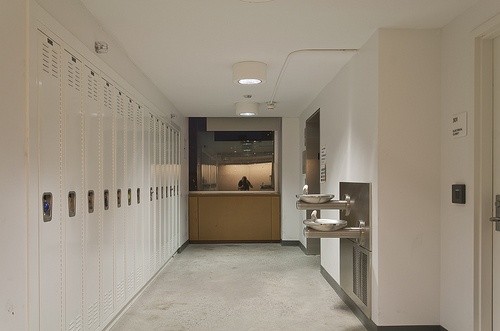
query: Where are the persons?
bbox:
[237,177,254,191]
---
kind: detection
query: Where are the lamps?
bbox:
[234,101,256,117]
[232,61,267,86]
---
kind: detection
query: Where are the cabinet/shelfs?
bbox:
[27,1,183,331]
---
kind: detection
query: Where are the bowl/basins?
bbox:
[296,194,335,204]
[303,218,348,232]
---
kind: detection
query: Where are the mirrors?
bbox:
[191,131,276,192]
